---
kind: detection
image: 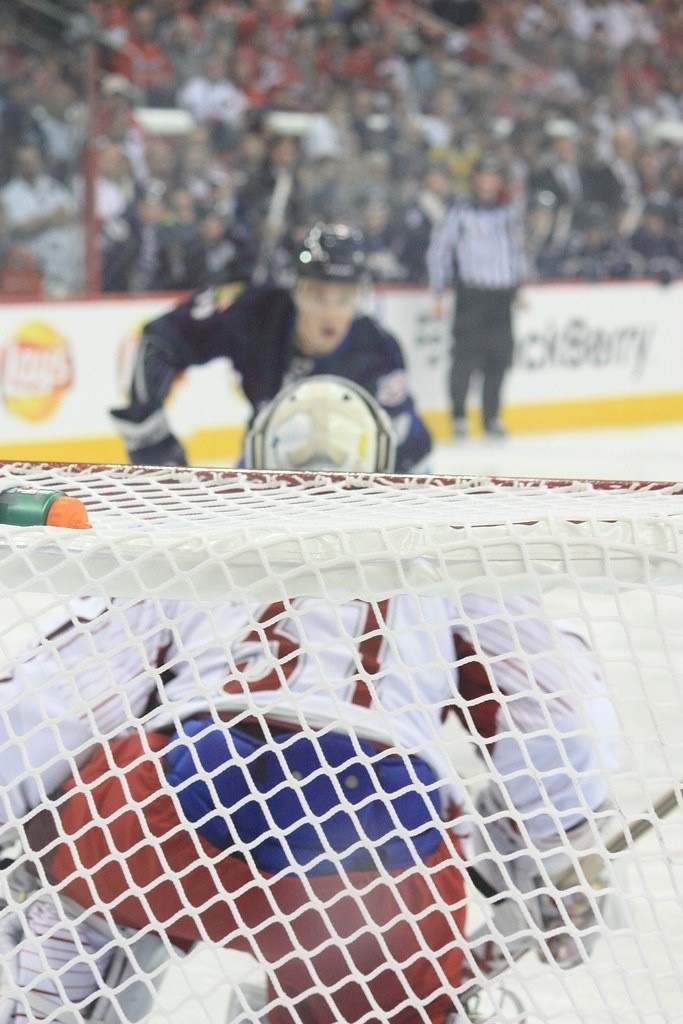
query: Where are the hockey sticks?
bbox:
[448,781,683,1007]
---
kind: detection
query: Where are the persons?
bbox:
[110,224,431,475]
[0,0,682,298]
[425,154,526,438]
[0,375,620,1024]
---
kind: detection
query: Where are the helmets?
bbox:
[280,213,366,274]
[473,154,510,177]
[245,374,397,472]
